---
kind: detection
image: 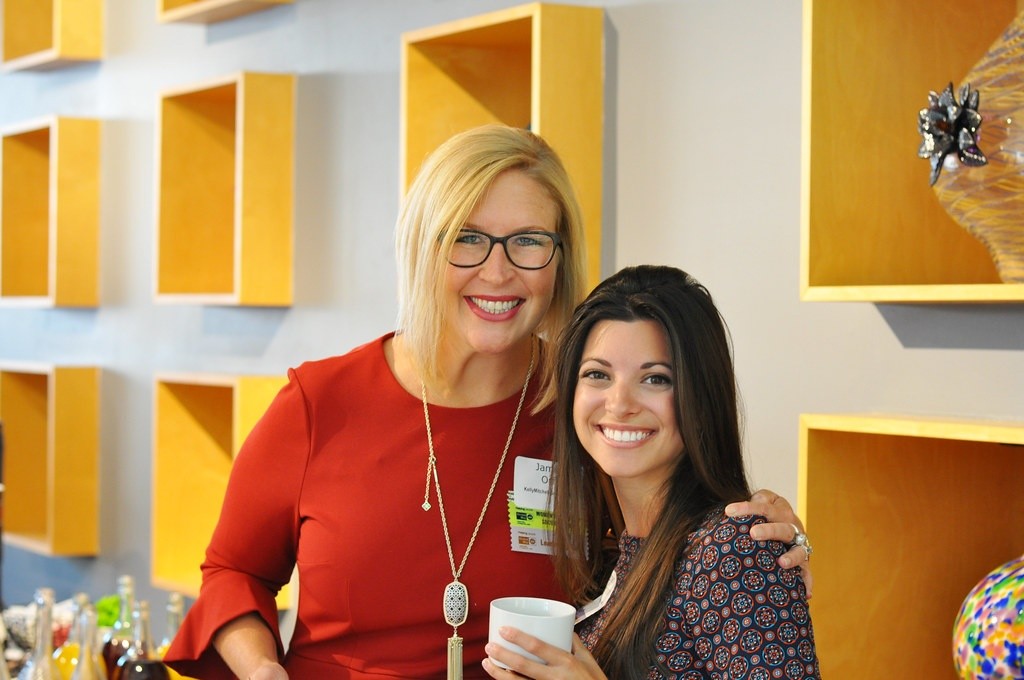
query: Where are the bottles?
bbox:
[17,575,183,680]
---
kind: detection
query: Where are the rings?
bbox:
[786,522,805,545]
[791,543,815,560]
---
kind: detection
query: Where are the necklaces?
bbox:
[420,331,540,680]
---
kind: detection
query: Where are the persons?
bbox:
[163,124,819,680]
[482,265,823,680]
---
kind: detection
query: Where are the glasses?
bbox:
[437,229,562,270]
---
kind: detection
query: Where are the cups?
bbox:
[489,597,576,670]
